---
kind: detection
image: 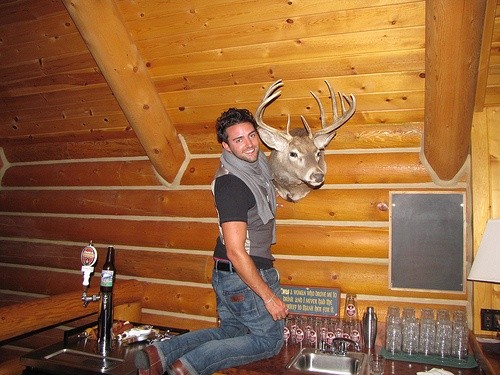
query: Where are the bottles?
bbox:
[78,321,157,344]
[361,306,378,349]
[100,245,116,291]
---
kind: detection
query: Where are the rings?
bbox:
[285,309,289,312]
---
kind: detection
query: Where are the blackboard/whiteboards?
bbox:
[388,190,467,295]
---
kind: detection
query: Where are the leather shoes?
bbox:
[166,358,192,375]
[134,345,163,375]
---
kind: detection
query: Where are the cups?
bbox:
[385,306,470,365]
[355,353,385,375]
[343,293,358,323]
[283,316,363,352]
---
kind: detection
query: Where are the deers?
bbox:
[252,77,357,202]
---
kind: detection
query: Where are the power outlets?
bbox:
[480,308,500,332]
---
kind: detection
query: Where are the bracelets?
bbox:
[265,292,276,304]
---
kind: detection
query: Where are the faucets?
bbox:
[81,291,101,308]
[333,337,361,357]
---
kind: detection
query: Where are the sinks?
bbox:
[285,346,367,375]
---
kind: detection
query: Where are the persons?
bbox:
[134,107,289,375]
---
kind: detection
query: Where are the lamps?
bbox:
[467,216,500,284]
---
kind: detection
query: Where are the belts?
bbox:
[214,260,236,272]
